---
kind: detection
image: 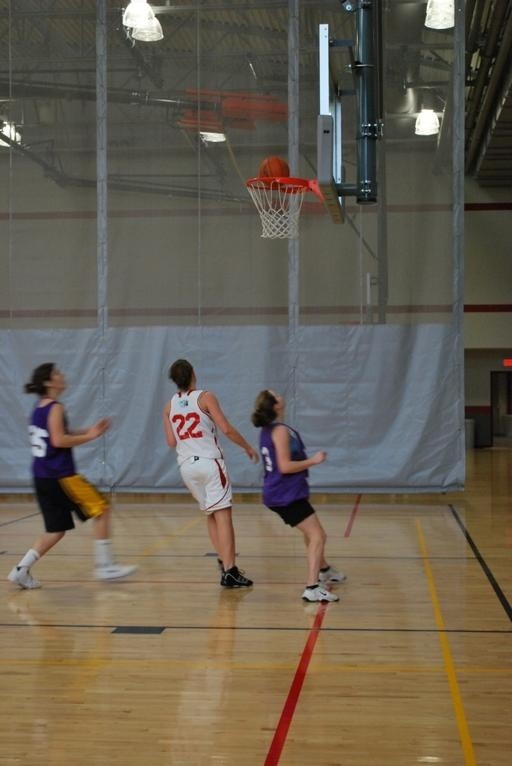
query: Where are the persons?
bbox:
[251,389,347,601]
[164,359,259,588]
[6,363,138,590]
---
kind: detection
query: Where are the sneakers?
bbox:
[93,562,138,579]
[319,567,347,583]
[7,566,41,588]
[217,558,253,586]
[301,586,340,602]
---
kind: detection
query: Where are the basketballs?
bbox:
[257,155,291,186]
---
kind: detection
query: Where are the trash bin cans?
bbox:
[465,418,476,449]
